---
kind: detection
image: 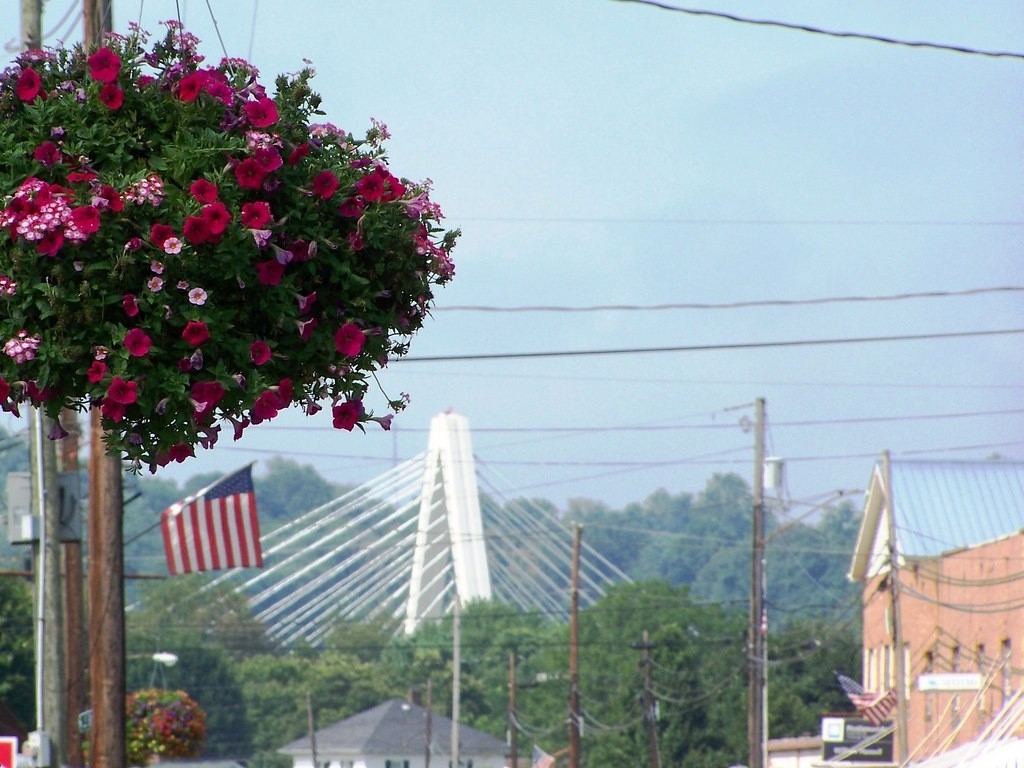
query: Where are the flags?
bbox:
[158,462,266,577]
[833,674,896,730]
